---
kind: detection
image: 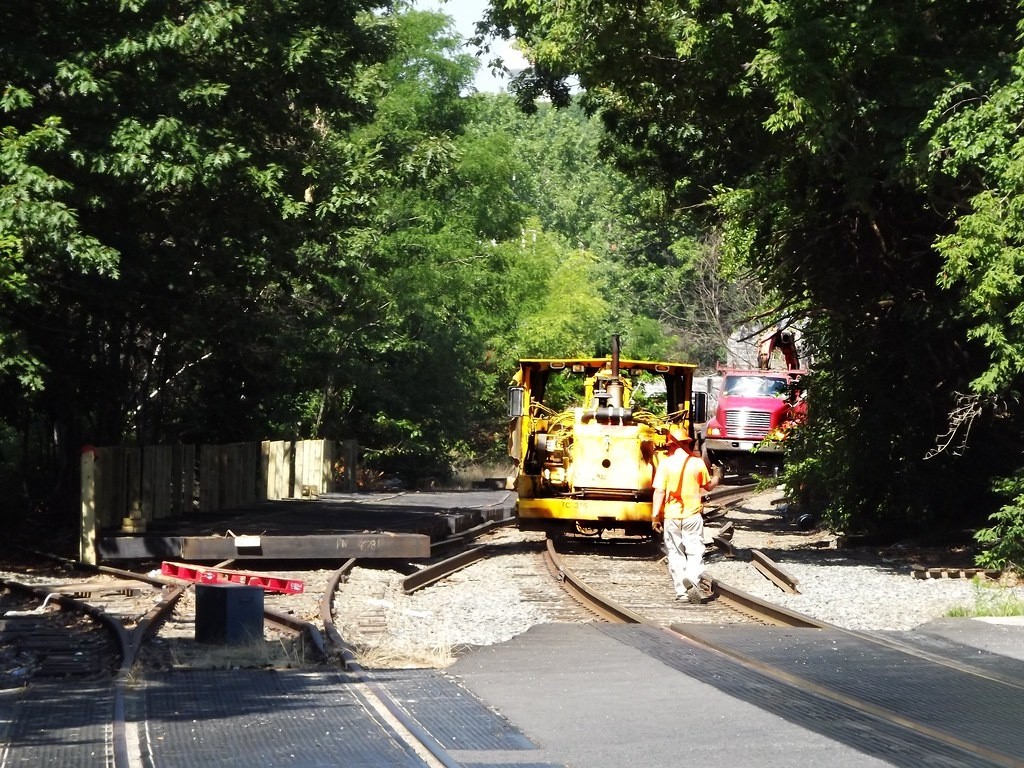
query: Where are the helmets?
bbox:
[665,428,692,445]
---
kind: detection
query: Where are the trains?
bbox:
[504,329,711,539]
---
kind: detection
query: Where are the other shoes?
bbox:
[674,596,688,602]
[683,578,701,604]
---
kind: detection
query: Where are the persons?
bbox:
[652,423,725,605]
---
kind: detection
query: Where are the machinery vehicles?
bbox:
[702,326,814,475]
